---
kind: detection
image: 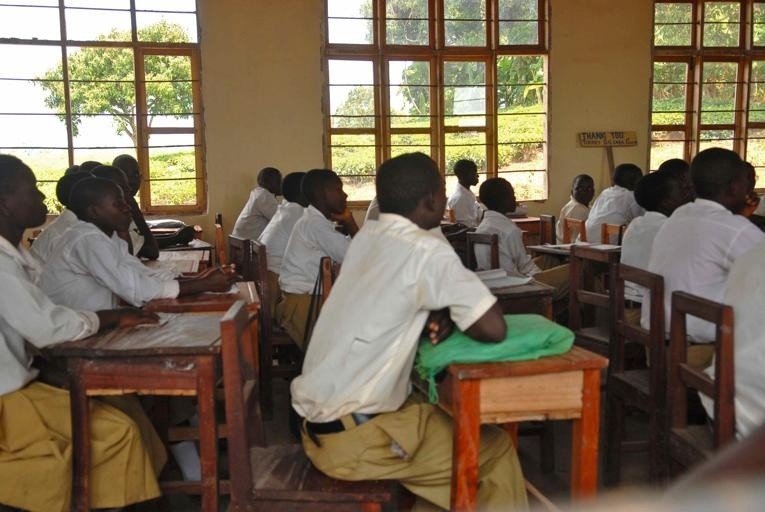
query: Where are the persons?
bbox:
[275,168,361,354]
[472,177,609,328]
[446,158,522,229]
[230,165,285,275]
[29,153,242,484]
[291,151,532,511]
[0,153,171,511]
[556,174,595,245]
[585,147,764,440]
[252,170,310,321]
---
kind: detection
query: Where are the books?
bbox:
[141,260,200,274]
[505,204,529,219]
[476,269,535,290]
[156,250,211,262]
[544,241,622,253]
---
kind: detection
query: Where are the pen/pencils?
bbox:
[214,262,239,287]
[180,222,197,240]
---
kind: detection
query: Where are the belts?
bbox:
[665,340,716,346]
[300,412,380,447]
[625,299,642,308]
